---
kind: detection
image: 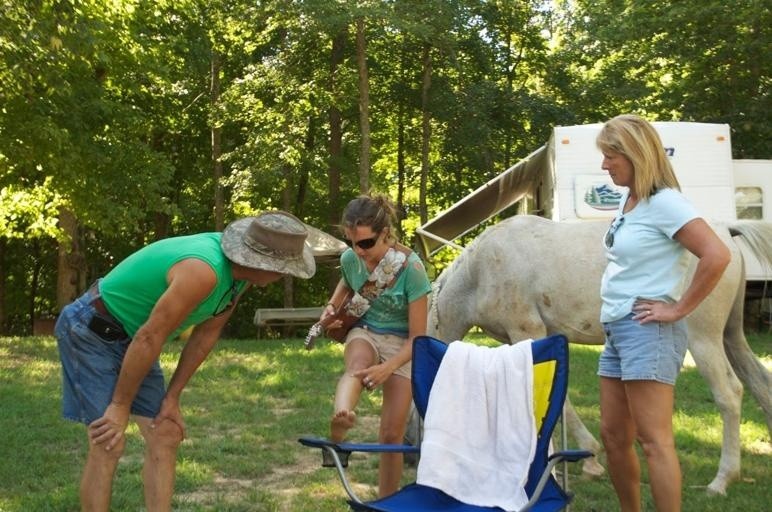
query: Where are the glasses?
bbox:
[342,228,384,248]
[605,218,623,247]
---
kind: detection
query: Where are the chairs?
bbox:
[299,334,593,512]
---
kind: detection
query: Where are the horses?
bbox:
[402,212,772,501]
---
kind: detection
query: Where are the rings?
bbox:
[647,310,650,315]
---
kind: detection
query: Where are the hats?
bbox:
[221,211,317,280]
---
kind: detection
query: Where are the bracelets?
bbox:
[325,303,336,311]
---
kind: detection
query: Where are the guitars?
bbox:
[303,290,357,350]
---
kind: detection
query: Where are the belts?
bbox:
[87,280,133,344]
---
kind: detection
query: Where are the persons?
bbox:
[595,114,731,512]
[53,212,316,512]
[319,195,433,498]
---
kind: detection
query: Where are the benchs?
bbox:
[252,307,324,340]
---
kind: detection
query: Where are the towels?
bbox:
[414,339,557,512]
